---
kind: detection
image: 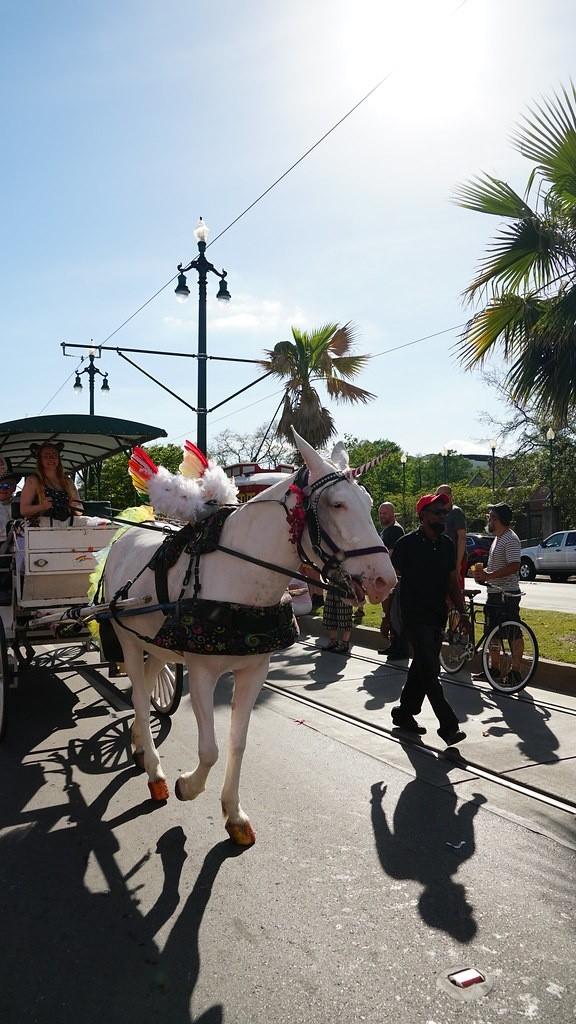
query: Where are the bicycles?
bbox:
[434,581,540,695]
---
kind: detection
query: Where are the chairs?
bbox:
[12,498,111,575]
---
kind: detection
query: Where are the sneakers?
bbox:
[471,665,501,681]
[494,671,523,687]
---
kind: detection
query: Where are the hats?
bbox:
[487,502,513,522]
[416,495,450,516]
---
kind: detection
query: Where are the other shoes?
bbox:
[332,640,348,652]
[391,712,428,735]
[441,723,466,741]
[378,647,393,655]
[322,638,339,651]
[386,650,409,661]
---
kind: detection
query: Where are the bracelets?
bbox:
[461,614,468,617]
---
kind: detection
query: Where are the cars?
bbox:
[517,530,576,582]
[463,532,496,578]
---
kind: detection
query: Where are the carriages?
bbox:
[0,414,396,844]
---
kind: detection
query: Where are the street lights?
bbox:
[545,427,555,534]
[400,454,407,532]
[174,214,232,460]
[490,437,497,492]
[73,338,110,498]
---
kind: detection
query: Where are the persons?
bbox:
[437,485,467,641]
[288,565,325,615]
[0,478,17,547]
[322,583,353,653]
[381,493,473,746]
[20,440,107,527]
[378,502,410,660]
[472,502,525,688]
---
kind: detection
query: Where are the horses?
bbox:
[100,424,400,848]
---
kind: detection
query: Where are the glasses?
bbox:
[0,484,16,489]
[424,509,449,516]
[41,454,59,460]
